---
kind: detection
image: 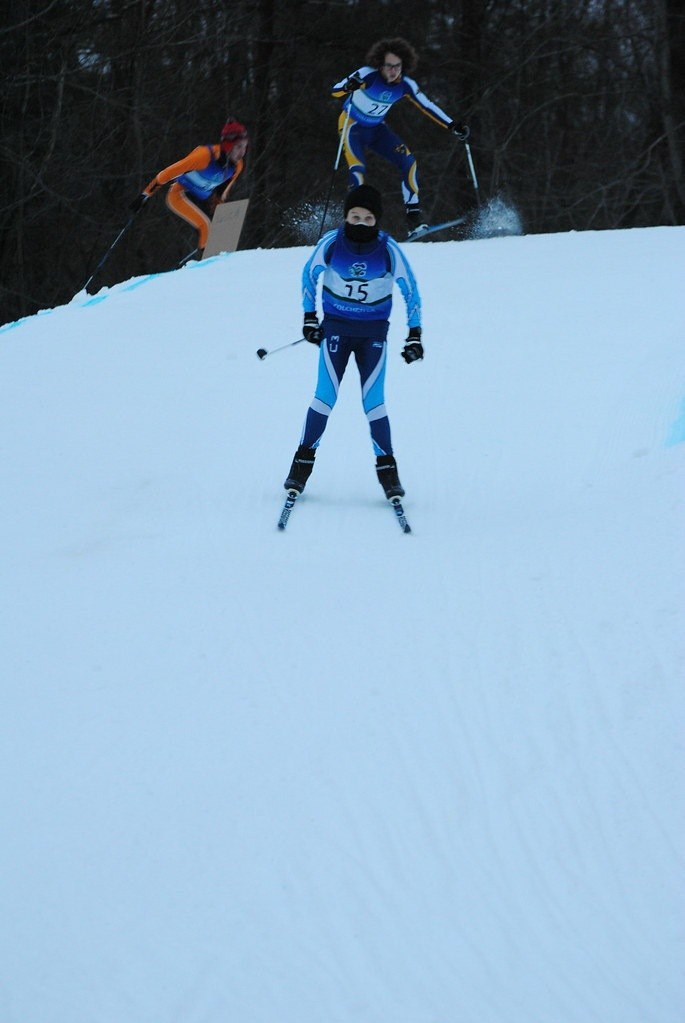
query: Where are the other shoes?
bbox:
[198,248,204,260]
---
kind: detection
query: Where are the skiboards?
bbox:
[176,248,205,270]
[276,494,412,533]
[405,216,466,242]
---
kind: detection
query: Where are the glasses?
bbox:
[384,63,403,71]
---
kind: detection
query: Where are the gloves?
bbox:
[129,194,150,214]
[303,311,324,345]
[448,120,471,141]
[343,72,365,93]
[401,326,423,364]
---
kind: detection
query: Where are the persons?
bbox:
[331,38,470,236]
[132,117,248,269]
[277,185,425,534]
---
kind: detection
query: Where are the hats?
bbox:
[220,116,249,181]
[344,185,382,224]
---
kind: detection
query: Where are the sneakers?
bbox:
[375,456,405,500]
[403,204,428,237]
[284,445,316,494]
